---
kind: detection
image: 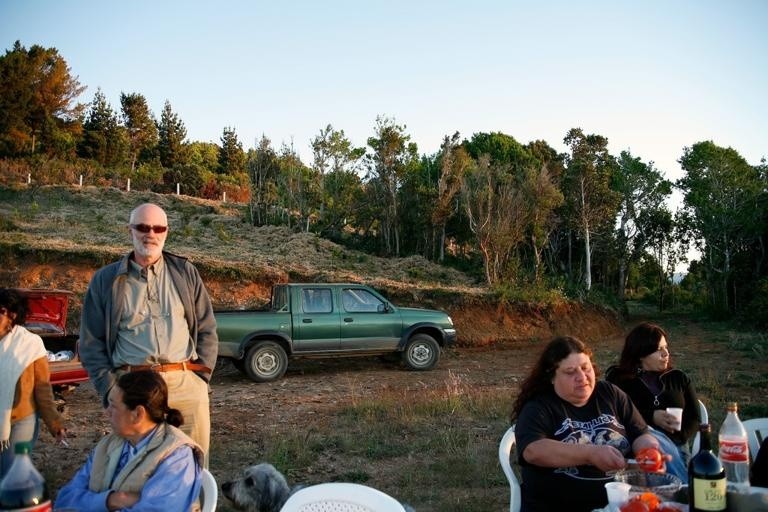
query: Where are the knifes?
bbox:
[624,459,657,465]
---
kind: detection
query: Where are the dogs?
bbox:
[221,462,419,512]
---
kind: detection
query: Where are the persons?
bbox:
[511,336,672,512]
[606,322,702,456]
[54,368,204,512]
[749,437,768,490]
[0,287,66,484]
[79,203,218,473]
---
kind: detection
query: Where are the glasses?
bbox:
[0,308,13,320]
[129,223,169,234]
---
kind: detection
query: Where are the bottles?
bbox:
[688,425,728,512]
[1,441,52,512]
[718,403,750,494]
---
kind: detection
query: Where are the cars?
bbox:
[5,286,90,385]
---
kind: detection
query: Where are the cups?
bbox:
[666,408,684,432]
[604,481,632,510]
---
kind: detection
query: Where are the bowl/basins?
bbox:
[614,471,683,502]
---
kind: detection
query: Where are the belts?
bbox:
[115,360,212,375]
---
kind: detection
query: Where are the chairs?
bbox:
[681,400,708,463]
[277,482,406,512]
[198,470,218,512]
[717,418,768,466]
[499,423,522,512]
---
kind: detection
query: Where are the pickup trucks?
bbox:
[214,281,456,382]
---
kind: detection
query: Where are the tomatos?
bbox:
[636,448,663,472]
[621,500,647,512]
